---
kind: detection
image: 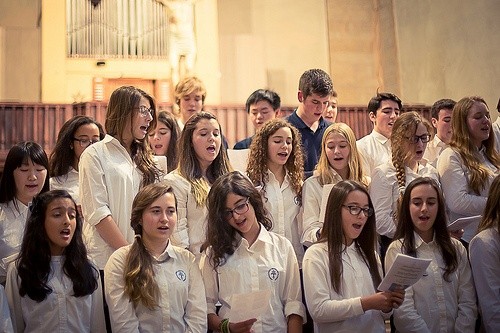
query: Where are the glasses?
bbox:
[222,196,250,221]
[74,136,99,148]
[134,105,153,117]
[343,204,374,217]
[404,134,431,144]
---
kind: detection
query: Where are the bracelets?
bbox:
[218,318,231,333]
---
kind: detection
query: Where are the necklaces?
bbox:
[153,256,170,264]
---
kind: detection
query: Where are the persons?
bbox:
[302,180,406,333]
[196,171,306,333]
[79,86,164,333]
[372,112,443,333]
[0,142,50,287]
[246,119,313,333]
[469,175,500,333]
[49,117,104,204]
[436,96,500,333]
[104,183,207,333]
[384,177,477,333]
[296,123,372,253]
[144,69,500,179]
[160,112,234,333]
[6,190,106,333]
